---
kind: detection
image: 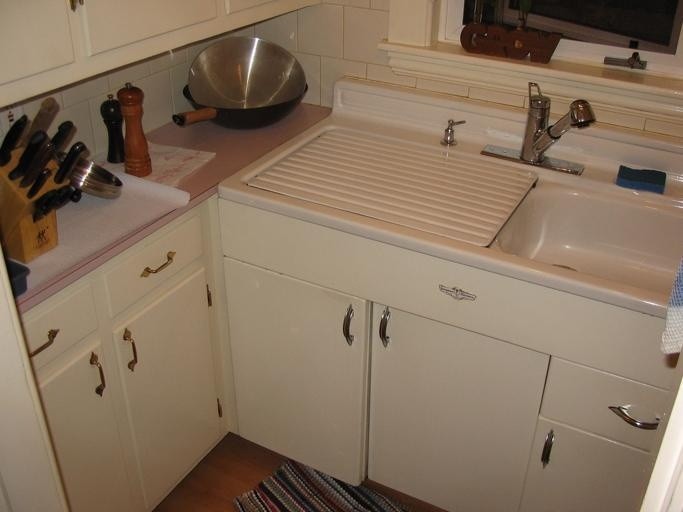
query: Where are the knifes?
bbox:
[2,97,86,224]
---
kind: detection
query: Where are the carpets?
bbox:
[227,461,407,512]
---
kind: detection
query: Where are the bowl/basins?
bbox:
[188,34,307,111]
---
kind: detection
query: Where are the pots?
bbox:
[173,84,310,131]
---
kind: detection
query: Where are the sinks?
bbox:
[494,179,683,296]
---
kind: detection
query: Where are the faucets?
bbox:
[480,82,597,177]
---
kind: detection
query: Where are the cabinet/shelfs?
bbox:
[0,0,328,105]
[217,195,682,512]
[17,201,227,511]
